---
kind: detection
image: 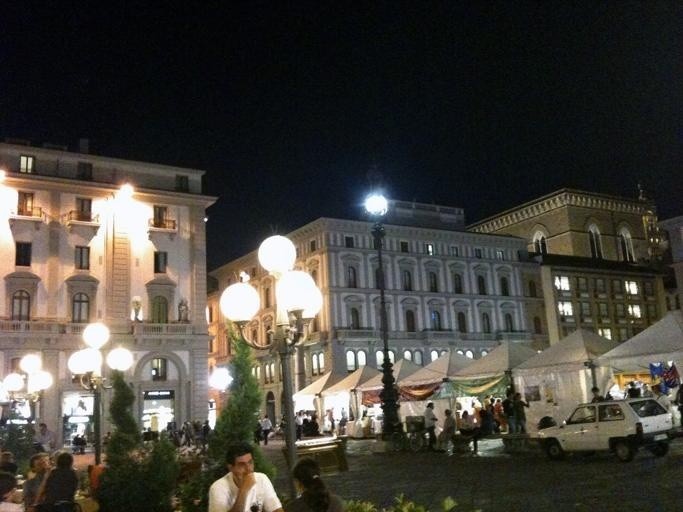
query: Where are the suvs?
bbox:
[538,395,675,466]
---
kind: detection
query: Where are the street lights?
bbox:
[0,351,57,426]
[209,231,327,501]
[361,189,408,456]
[65,320,137,466]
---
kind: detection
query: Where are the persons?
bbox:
[207,439,285,512]
[421,388,530,453]
[290,406,348,442]
[1,422,110,510]
[589,380,683,426]
[287,455,345,511]
[139,420,213,456]
[250,412,273,447]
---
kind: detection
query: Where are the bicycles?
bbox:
[388,419,425,454]
